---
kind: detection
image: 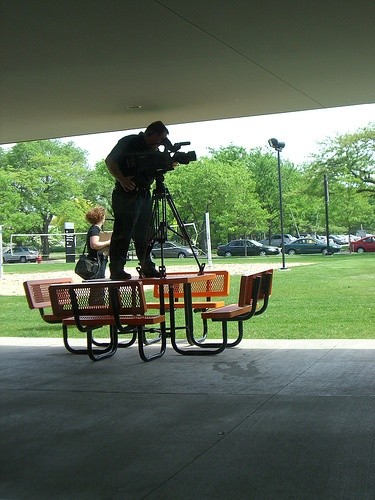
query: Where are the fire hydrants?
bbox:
[36,256,42,263]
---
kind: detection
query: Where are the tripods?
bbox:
[138,170,204,279]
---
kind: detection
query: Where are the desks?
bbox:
[82,273,215,356]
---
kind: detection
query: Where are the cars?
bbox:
[261,234,361,247]
[150,241,198,259]
[217,240,280,258]
[281,238,341,255]
[350,235,375,254]
[3,246,38,263]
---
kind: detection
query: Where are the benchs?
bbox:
[23,269,273,363]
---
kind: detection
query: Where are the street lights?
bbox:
[268,138,287,270]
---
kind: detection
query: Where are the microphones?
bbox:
[180,142,191,145]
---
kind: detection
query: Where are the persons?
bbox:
[85,206,110,306]
[105,121,177,280]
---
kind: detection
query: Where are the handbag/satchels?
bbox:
[75,254,101,280]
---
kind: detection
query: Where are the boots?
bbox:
[138,263,166,279]
[109,265,132,280]
